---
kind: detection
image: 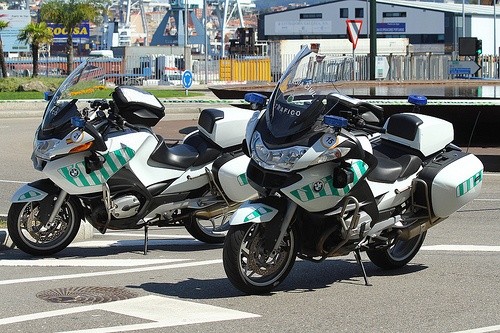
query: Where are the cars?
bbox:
[158,73,184,86]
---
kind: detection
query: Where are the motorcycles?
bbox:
[7,59,270,258]
[222,46,485,296]
